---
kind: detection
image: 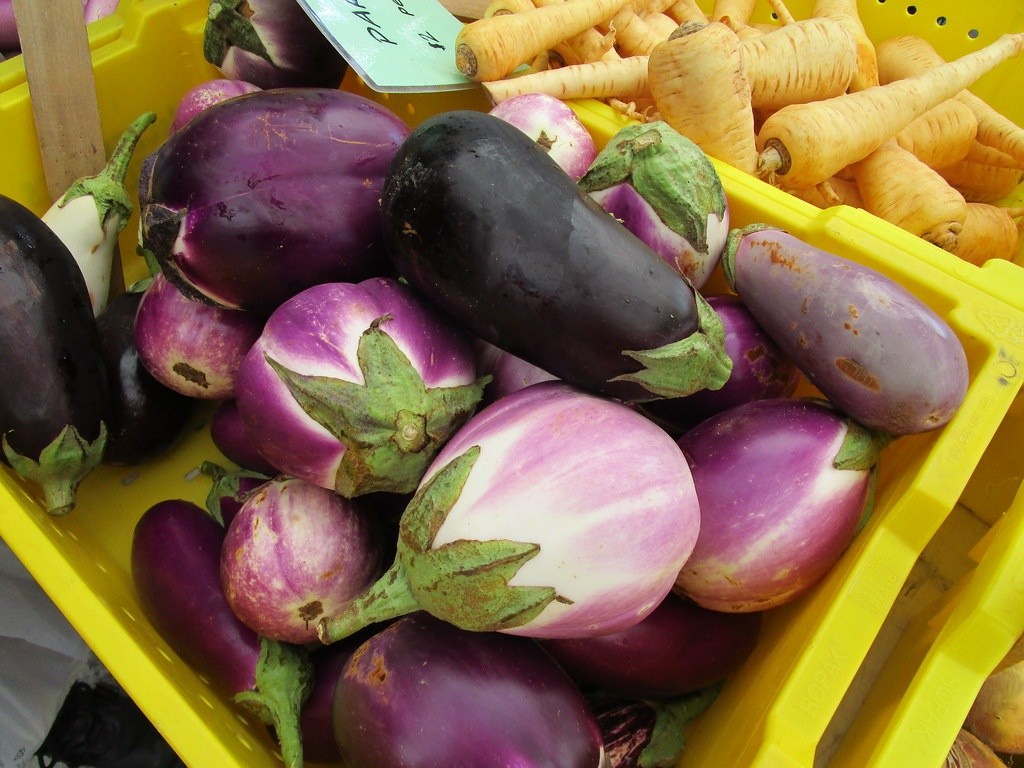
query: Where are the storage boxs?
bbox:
[0,0,1024,768]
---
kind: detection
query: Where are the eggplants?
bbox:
[0,76,968,768]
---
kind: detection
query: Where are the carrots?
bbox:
[453,0,1024,269]
